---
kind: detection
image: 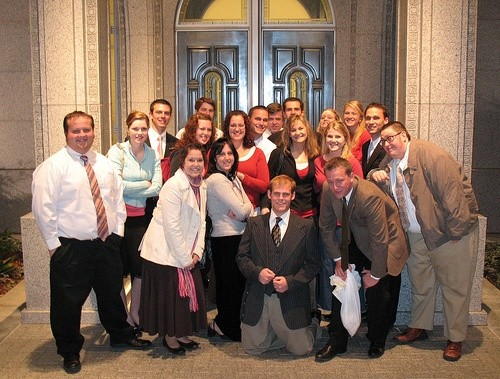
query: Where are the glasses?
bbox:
[379,132,402,147]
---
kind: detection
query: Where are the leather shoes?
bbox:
[443,342,463,361]
[110,336,152,350]
[368,343,385,358]
[393,328,428,343]
[62,354,81,374]
[316,343,347,362]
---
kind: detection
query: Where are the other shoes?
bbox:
[311,309,321,326]
[131,322,150,338]
[179,340,200,348]
[163,337,186,354]
[208,318,216,337]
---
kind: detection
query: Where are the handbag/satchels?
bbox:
[333,269,361,337]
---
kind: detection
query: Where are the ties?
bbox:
[272,216,283,250]
[394,164,411,231]
[341,196,349,272]
[80,155,110,241]
[367,142,374,163]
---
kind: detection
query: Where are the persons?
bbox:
[365,121,479,360]
[235,174,324,356]
[31,111,151,374]
[136,143,209,356]
[106,97,389,338]
[203,137,254,341]
[315,159,408,362]
